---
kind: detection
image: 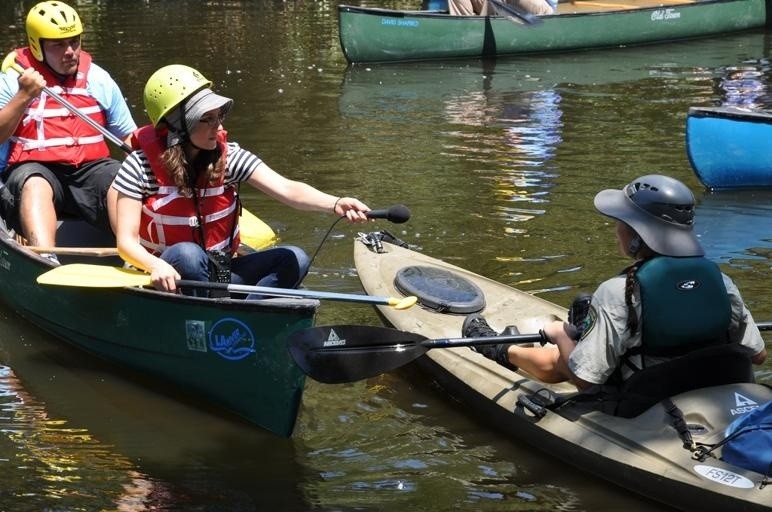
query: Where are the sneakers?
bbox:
[462,313,518,371]
[568,293,592,341]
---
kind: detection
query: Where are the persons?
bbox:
[0,0,142,265]
[446,1,556,18]
[461,173,767,409]
[112,62,377,300]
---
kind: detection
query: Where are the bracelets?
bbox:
[333,199,341,214]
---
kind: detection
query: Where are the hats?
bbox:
[165,84,234,148]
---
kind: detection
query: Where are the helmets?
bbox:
[143,64,214,128]
[26,0,83,62]
[593,174,705,258]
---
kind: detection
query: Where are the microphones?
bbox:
[363,204,411,224]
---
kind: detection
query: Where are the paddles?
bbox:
[0,50,281,254]
[285,322,579,386]
[34,262,418,313]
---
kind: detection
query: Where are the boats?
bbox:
[683,104,771,188]
[0,216,323,440]
[335,0,771,65]
[690,189,772,266]
[350,234,772,512]
[337,31,769,114]
[0,317,295,508]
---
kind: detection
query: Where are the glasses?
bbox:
[200,111,226,126]
